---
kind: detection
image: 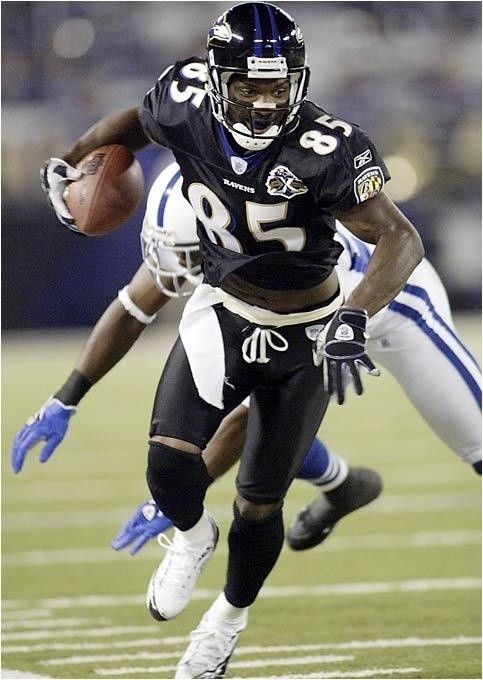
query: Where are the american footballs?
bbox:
[62,144,144,236]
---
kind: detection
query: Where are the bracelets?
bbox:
[48,369,93,410]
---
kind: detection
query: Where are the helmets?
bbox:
[208,5,311,154]
[138,161,214,288]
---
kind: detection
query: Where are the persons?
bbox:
[9,155,482,556]
[35,1,427,679]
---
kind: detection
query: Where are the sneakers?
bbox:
[285,467,383,550]
[172,589,249,678]
[143,513,220,623]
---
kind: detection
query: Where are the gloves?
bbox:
[9,394,80,474]
[311,302,383,404]
[109,499,175,556]
[36,155,86,237]
